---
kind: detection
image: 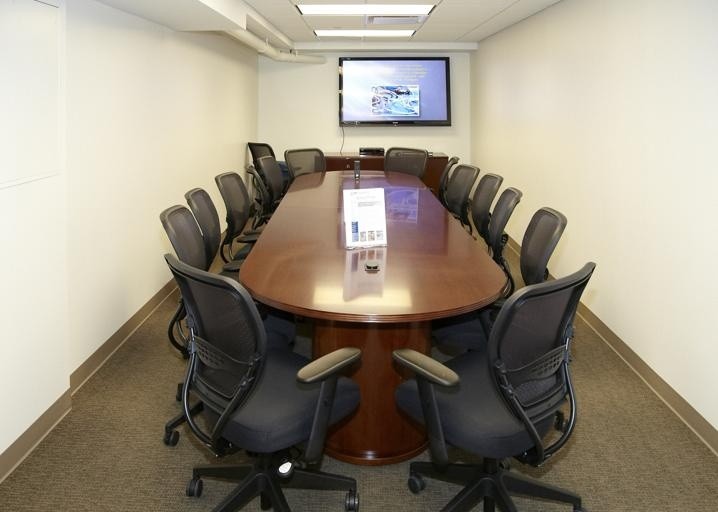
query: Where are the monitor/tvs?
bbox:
[339,57,451,126]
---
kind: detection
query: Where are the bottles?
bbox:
[359,249,384,261]
[359,230,383,244]
[354,161,361,177]
[351,213,359,242]
[350,252,358,274]
[355,178,360,186]
